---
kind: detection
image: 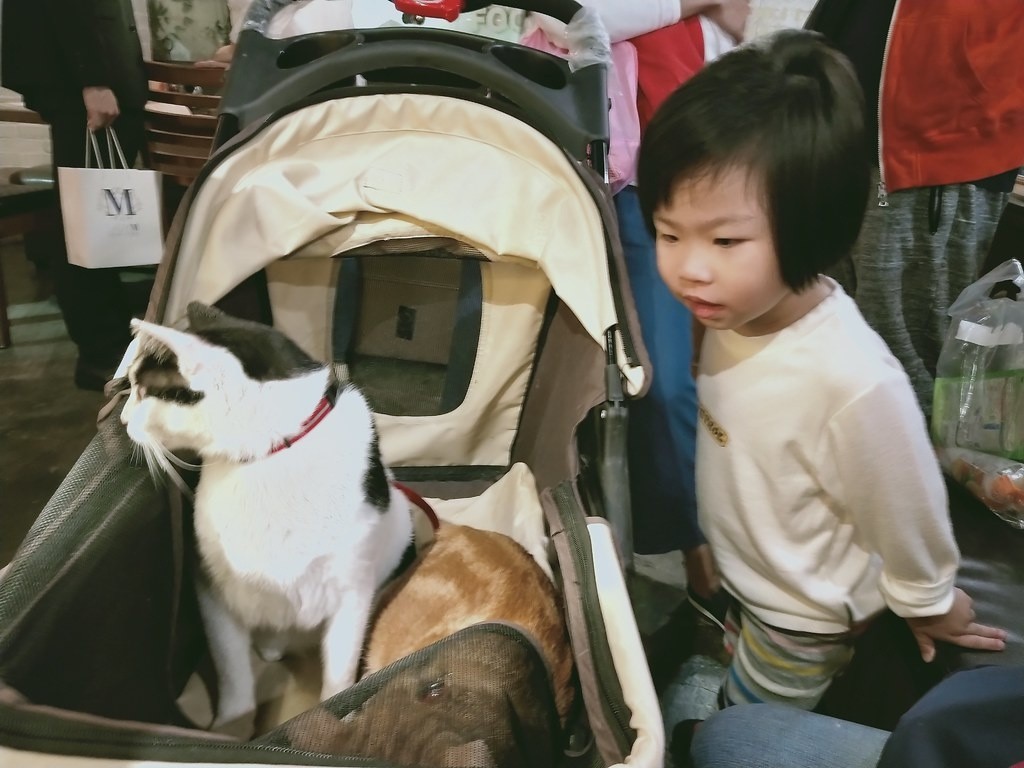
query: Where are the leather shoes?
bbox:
[74,351,125,390]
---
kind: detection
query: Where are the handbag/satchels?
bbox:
[59,123,164,269]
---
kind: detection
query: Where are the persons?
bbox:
[576,1,1024,768]
[0,0,152,392]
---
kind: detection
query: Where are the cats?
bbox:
[121,302,577,768]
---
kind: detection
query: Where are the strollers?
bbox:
[0,1,670,765]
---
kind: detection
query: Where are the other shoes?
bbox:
[685,583,728,632]
